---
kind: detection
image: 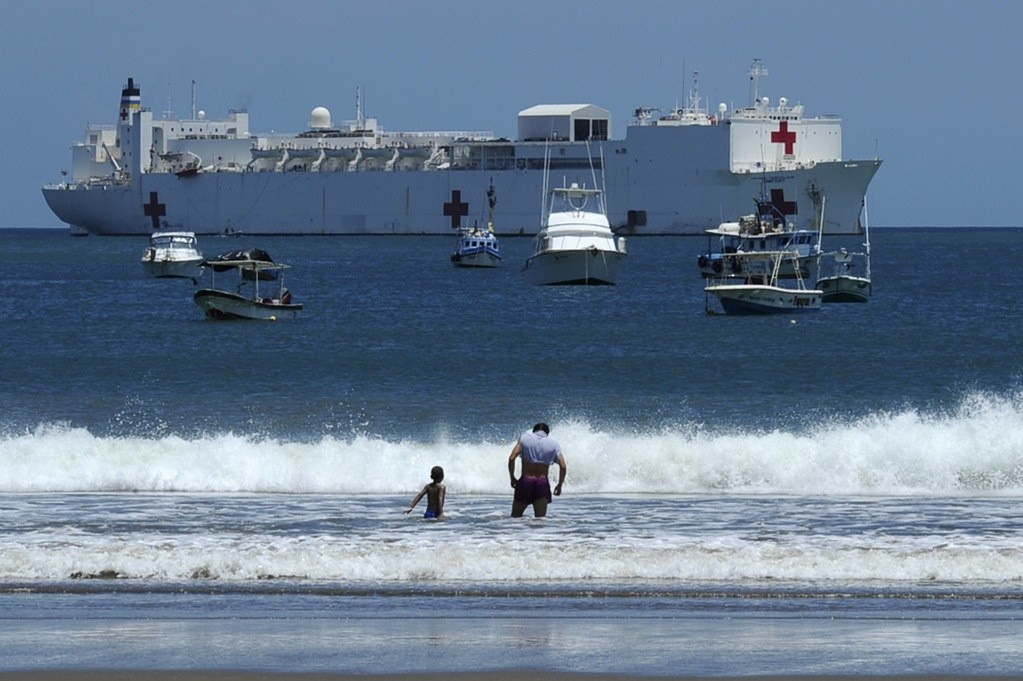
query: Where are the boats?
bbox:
[452,221,501,267]
[705,250,824,315]
[140,230,203,280]
[815,196,875,302]
[40,56,885,236]
[194,247,303,320]
[528,135,625,287]
[698,168,815,279]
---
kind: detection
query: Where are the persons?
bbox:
[469,227,491,237]
[403,466,446,520]
[508,422,567,517]
